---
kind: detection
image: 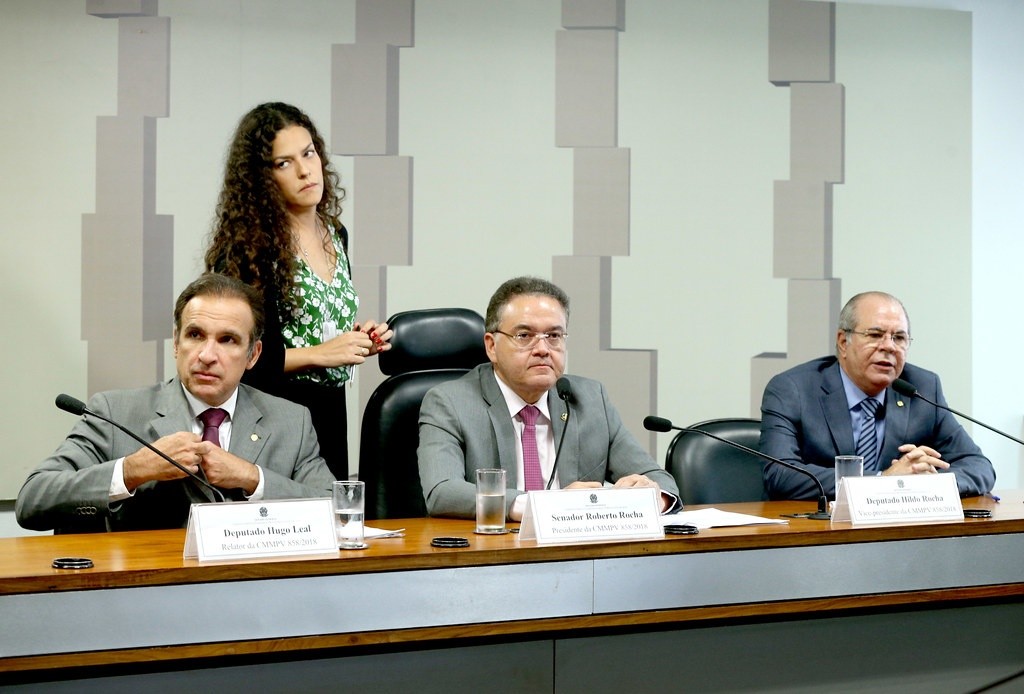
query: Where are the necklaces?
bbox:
[287,218,332,279]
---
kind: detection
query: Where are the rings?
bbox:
[360,348,364,355]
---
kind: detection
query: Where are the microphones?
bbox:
[55,393,227,503]
[643,416,832,520]
[892,379,1024,446]
[544,377,571,490]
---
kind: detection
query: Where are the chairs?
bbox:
[662,419,770,505]
[358,306,497,519]
[251,367,349,494]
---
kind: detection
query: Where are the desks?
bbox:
[0,495,1024,694]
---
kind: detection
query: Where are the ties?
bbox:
[197,407,228,449]
[856,398,880,472]
[517,405,544,493]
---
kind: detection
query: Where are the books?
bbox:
[658,507,789,529]
[335,523,405,539]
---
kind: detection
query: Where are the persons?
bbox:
[16,275,345,531]
[417,277,684,522]
[203,102,394,491]
[758,291,996,499]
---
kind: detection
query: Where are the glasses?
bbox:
[842,328,914,351]
[491,330,569,350]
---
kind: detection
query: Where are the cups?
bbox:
[835,455,863,501]
[332,481,365,549]
[476,469,507,534]
